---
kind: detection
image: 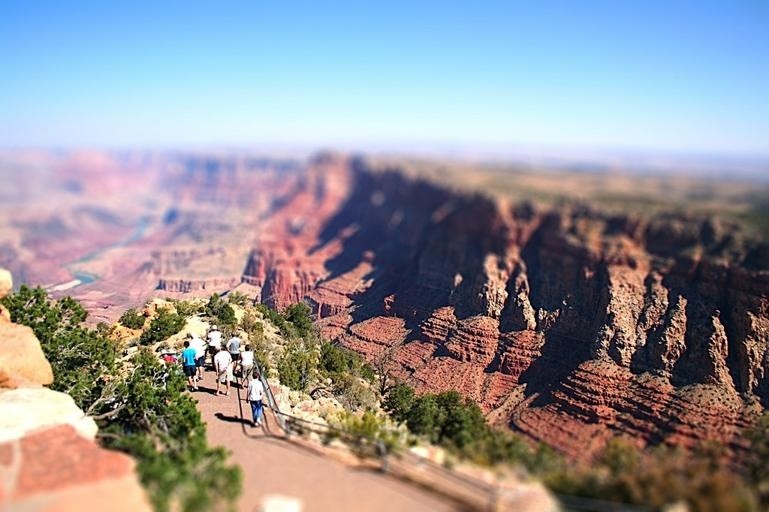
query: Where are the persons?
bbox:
[246,371,264,427]
[213,345,233,396]
[206,324,222,371]
[238,344,253,389]
[182,332,205,381]
[225,331,243,373]
[182,340,199,391]
[159,349,178,364]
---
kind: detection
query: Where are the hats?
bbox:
[211,325,218,329]
[159,350,168,358]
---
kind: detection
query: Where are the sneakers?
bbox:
[242,384,248,388]
[191,376,203,391]
[214,392,231,396]
[255,417,261,426]
